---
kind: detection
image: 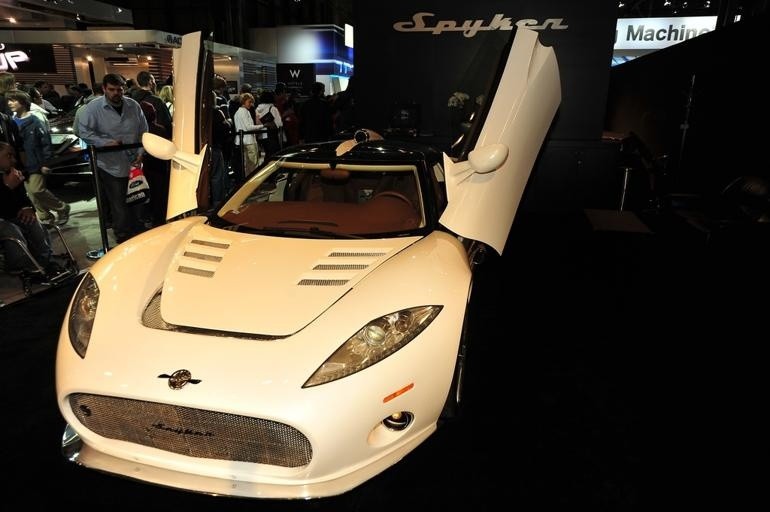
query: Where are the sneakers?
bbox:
[39,205,70,231]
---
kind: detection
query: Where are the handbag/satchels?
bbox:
[258,105,274,126]
[124,162,152,205]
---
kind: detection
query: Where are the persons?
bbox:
[211,75,355,198]
[0,71,174,285]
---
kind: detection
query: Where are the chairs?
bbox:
[299,172,359,204]
[371,170,422,218]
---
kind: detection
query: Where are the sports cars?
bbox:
[53,26,562,501]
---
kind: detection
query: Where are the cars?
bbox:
[45,121,107,197]
